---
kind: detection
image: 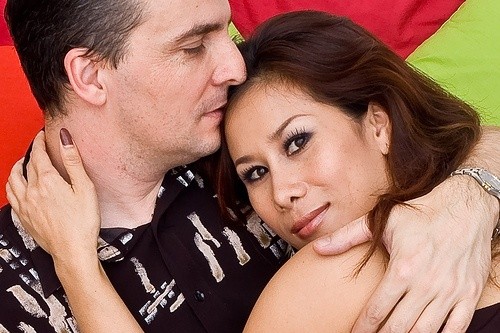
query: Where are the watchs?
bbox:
[449,166,500,241]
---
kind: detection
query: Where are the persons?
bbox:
[7,8,500,333]
[0,0,500,333]
